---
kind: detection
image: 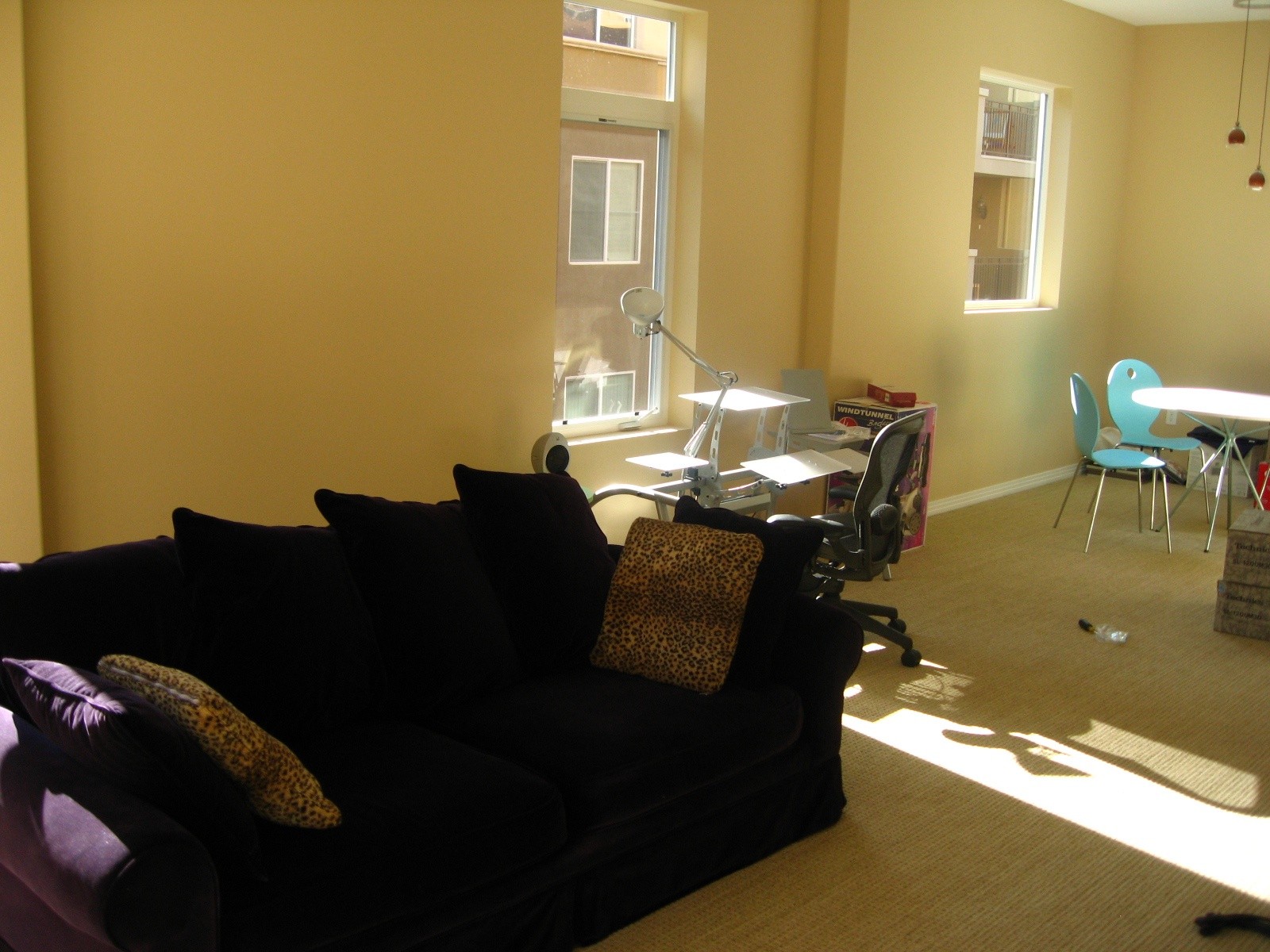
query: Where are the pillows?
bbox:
[6,463,821,831]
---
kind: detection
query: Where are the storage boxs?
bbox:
[1185,436,1268,499]
[1214,463,1270,640]
[867,382,918,407]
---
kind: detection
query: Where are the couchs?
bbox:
[0,526,862,952]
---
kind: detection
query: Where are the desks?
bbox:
[1132,388,1270,549]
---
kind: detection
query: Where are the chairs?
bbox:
[768,410,920,666]
[1052,358,1212,554]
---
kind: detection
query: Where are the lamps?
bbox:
[530,431,677,508]
[621,287,734,454]
[1228,0,1270,190]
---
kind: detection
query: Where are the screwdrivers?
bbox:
[1078,618,1105,639]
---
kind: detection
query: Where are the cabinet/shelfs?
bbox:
[824,396,937,553]
